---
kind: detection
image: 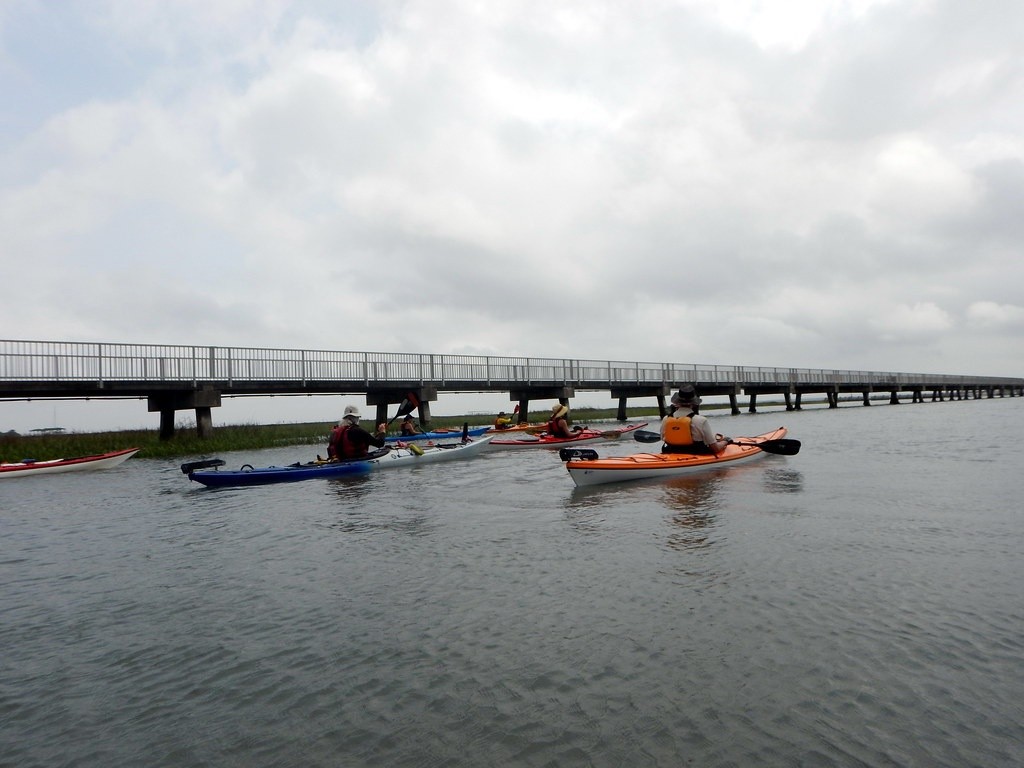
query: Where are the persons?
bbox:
[660,383,733,455]
[495,412,515,429]
[327,406,386,462]
[551,404,581,438]
[400,414,421,436]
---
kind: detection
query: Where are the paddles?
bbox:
[631,428,803,458]
[523,426,624,442]
[410,415,433,441]
[510,404,521,421]
[374,390,424,436]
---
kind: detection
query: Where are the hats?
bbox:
[342,405,361,419]
[403,414,414,422]
[497,411,507,416]
[551,404,568,419]
[670,384,702,406]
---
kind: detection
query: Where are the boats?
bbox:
[180,435,495,490]
[0,447,141,479]
[465,422,650,454]
[385,425,491,443]
[487,421,548,434]
[558,426,789,488]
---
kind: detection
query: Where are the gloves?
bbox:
[723,436,733,445]
